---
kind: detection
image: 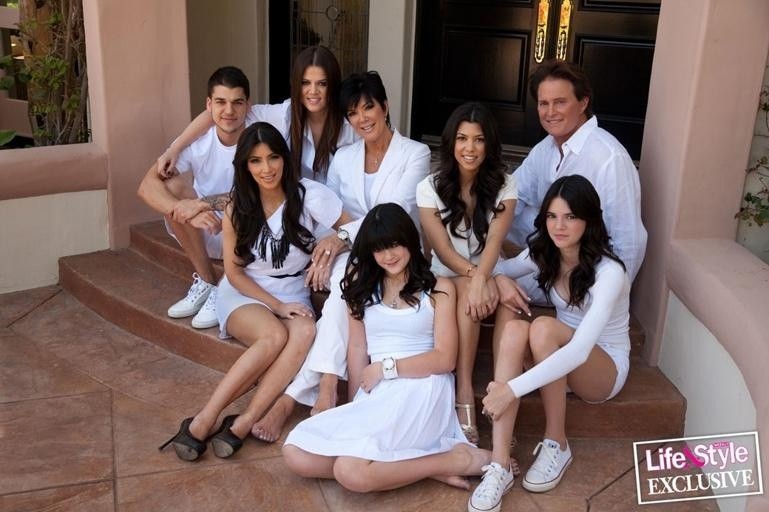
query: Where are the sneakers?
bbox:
[191,287,220,328]
[168,280,214,318]
[523,440,573,493]
[468,462,514,512]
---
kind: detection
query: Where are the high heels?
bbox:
[159,418,207,462]
[208,414,243,458]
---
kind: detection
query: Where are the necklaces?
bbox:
[365,141,388,166]
[383,282,406,308]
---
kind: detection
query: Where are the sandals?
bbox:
[454,403,478,447]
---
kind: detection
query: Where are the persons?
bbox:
[157,121,352,463]
[488,58,651,311]
[135,66,256,331]
[277,199,520,496]
[414,101,520,453]
[249,71,432,447]
[465,174,633,512]
[154,44,359,189]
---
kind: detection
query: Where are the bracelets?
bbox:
[336,229,349,247]
[382,357,399,381]
[466,265,478,277]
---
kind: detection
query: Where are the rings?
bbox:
[325,250,331,256]
